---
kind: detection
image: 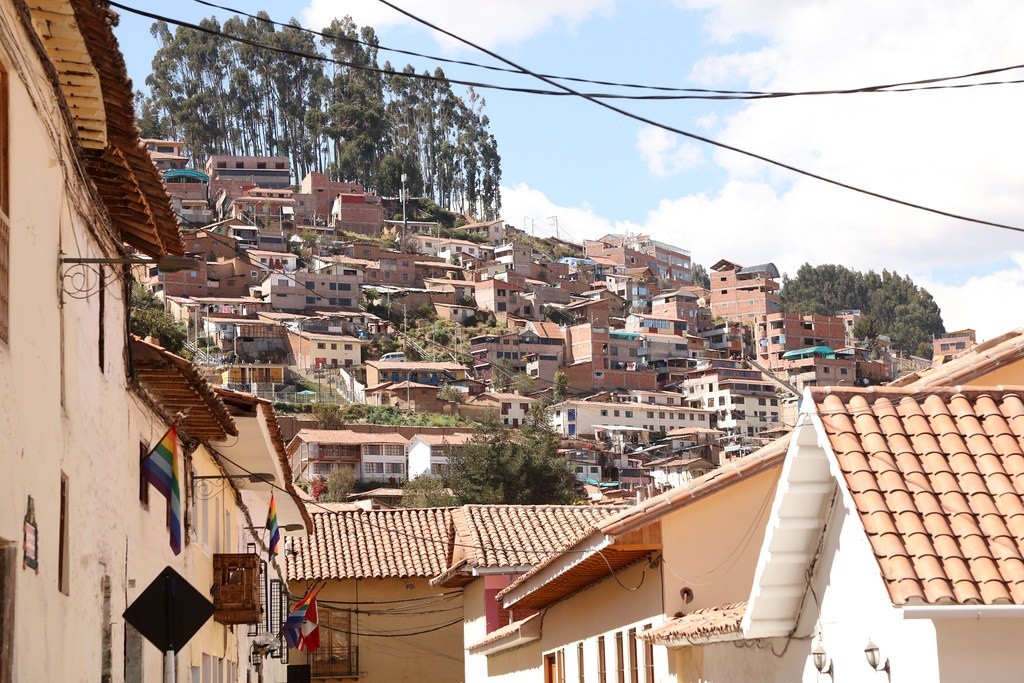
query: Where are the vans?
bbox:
[378,351,406,362]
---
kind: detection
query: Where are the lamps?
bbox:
[864,636,890,674]
[249,632,281,665]
[190,471,275,506]
[244,524,304,531]
[812,642,833,677]
[64,257,200,273]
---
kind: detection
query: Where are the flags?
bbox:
[266,491,280,562]
[279,579,326,654]
[141,419,181,556]
